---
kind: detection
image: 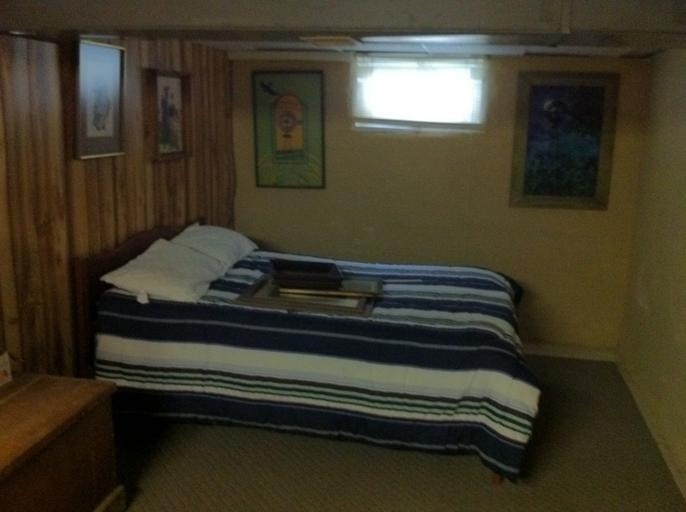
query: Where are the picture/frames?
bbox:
[252,70,325,189]
[509,71,621,210]
[74,39,127,160]
[230,259,383,318]
[151,68,193,164]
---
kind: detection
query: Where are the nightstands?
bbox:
[0,371,128,511]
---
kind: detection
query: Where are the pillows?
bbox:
[99,238,226,304]
[170,222,259,272]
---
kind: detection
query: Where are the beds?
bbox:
[74,216,542,484]
